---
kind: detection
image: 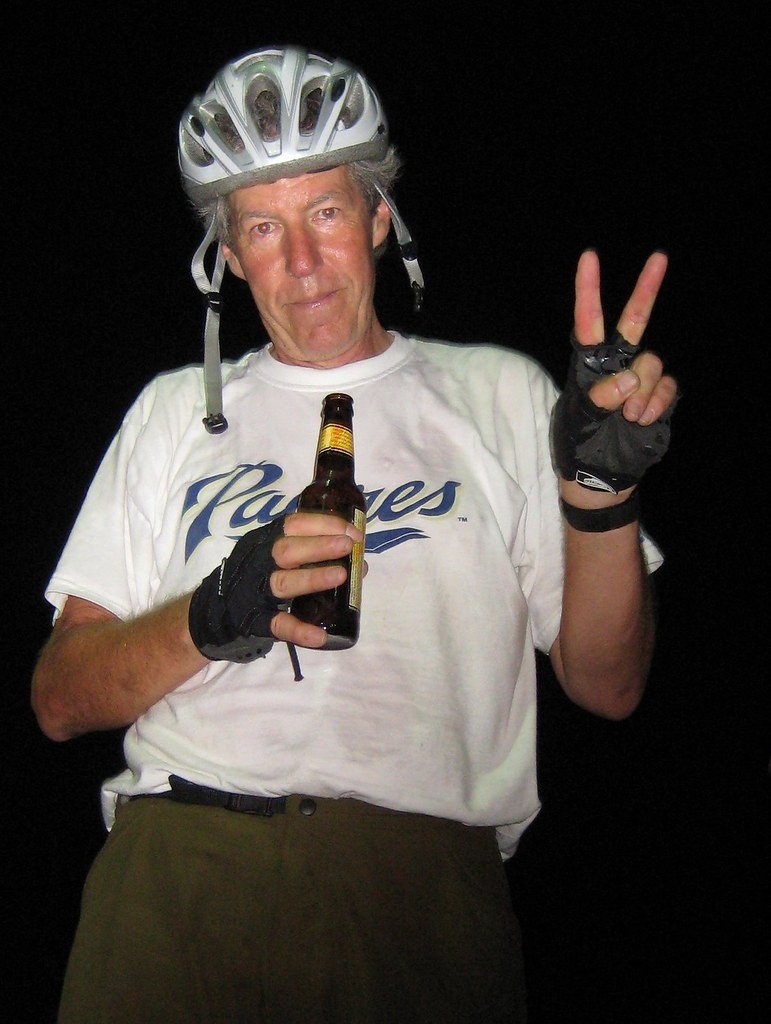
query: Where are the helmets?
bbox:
[175,43,391,208]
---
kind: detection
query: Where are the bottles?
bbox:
[291,393,366,650]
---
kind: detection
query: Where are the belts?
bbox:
[128,778,286,817]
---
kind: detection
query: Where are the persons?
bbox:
[30,48,683,1024]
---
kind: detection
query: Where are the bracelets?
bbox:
[558,486,640,533]
